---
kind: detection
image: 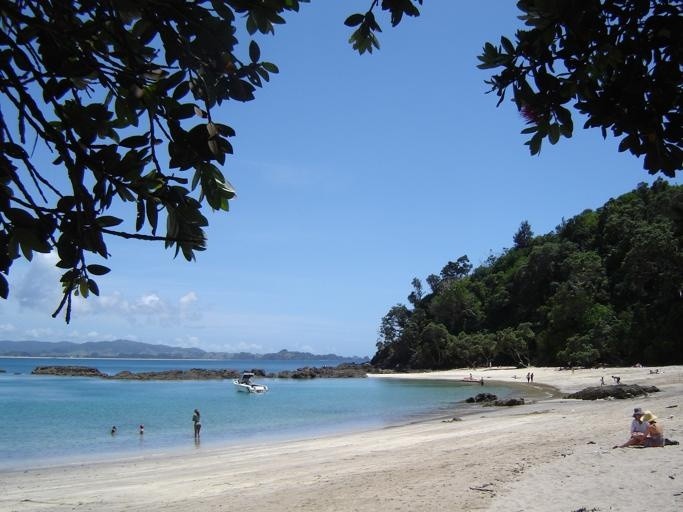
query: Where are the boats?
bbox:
[234,373,269,395]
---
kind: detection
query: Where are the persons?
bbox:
[139,424,144,435]
[611,376,620,385]
[111,425,116,432]
[191,408,201,438]
[531,372,534,383]
[600,377,607,387]
[616,411,665,448]
[527,373,530,383]
[630,407,679,445]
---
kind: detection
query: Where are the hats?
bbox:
[640,411,658,423]
[632,408,644,416]
